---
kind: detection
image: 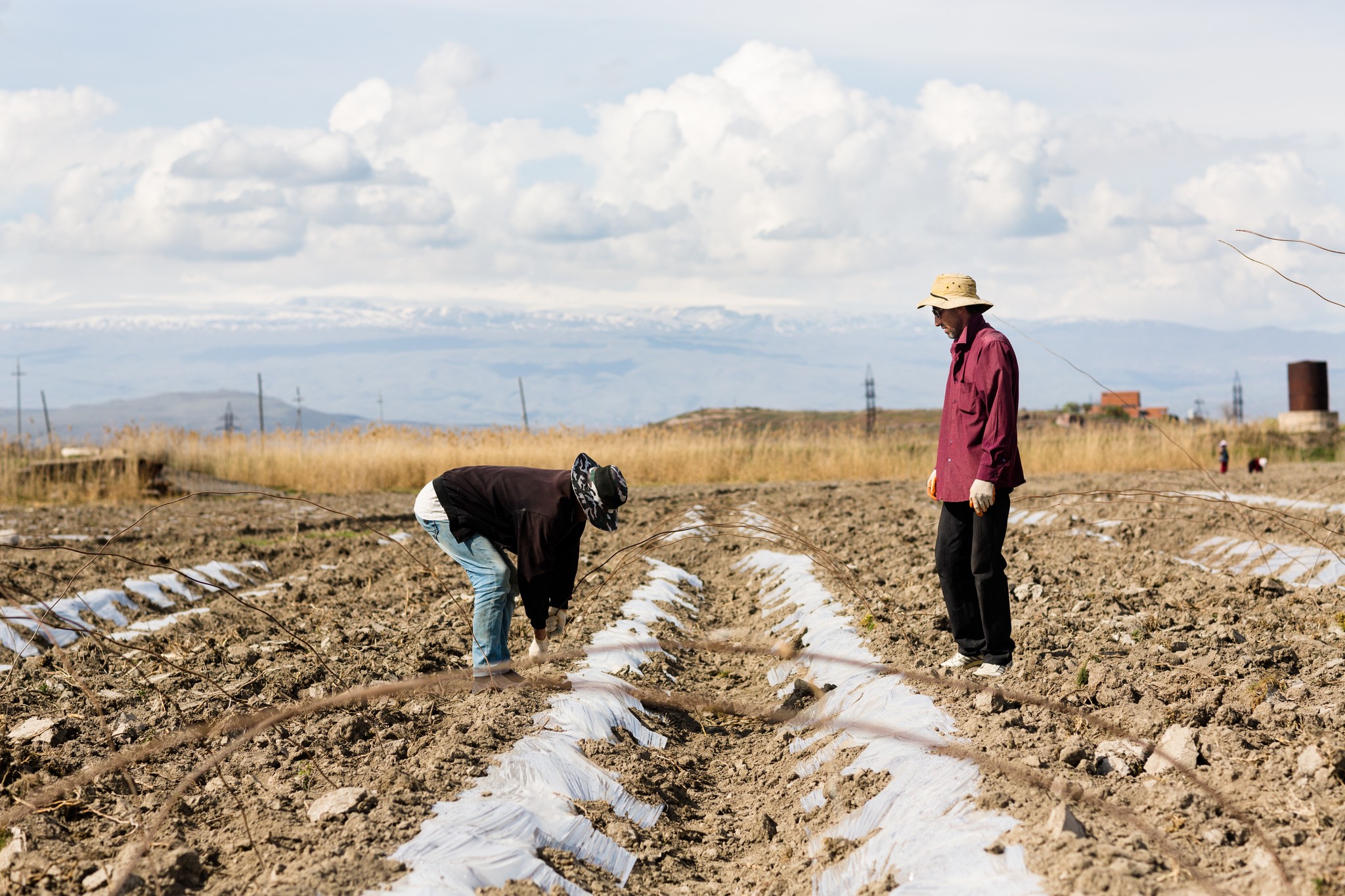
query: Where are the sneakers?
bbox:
[940,652,983,671]
[972,660,1013,677]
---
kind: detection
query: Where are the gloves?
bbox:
[926,469,941,502]
[546,607,566,638]
[528,635,548,666]
[969,479,995,517]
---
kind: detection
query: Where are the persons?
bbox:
[1248,457,1268,473]
[916,273,1028,679]
[1218,440,1229,473]
[414,454,628,694]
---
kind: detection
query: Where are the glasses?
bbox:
[932,308,942,319]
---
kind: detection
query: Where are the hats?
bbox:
[571,452,629,532]
[917,274,994,314]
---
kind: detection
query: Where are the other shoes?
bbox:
[471,671,531,694]
